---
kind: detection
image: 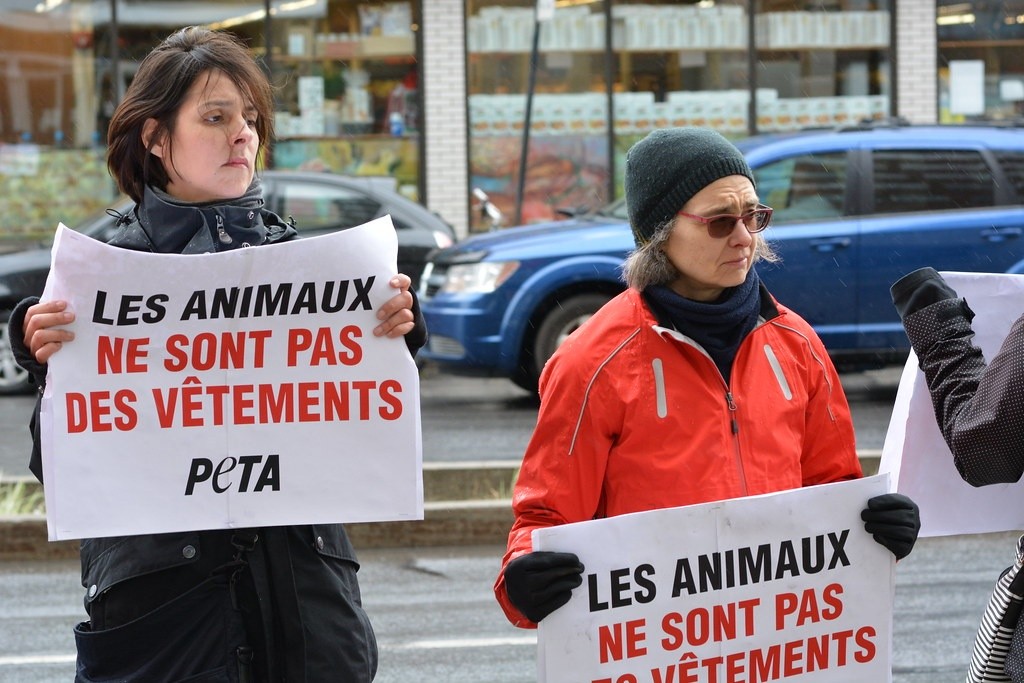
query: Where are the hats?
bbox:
[624,126,757,251]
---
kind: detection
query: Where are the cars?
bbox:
[0,168,463,399]
[418,107,1024,400]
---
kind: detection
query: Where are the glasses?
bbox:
[677,203,774,239]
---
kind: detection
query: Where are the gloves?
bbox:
[861,493,921,559]
[503,550,585,624]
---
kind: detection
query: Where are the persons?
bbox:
[890,267,1024,683]
[494,128,921,628]
[770,160,843,220]
[9,27,427,683]
